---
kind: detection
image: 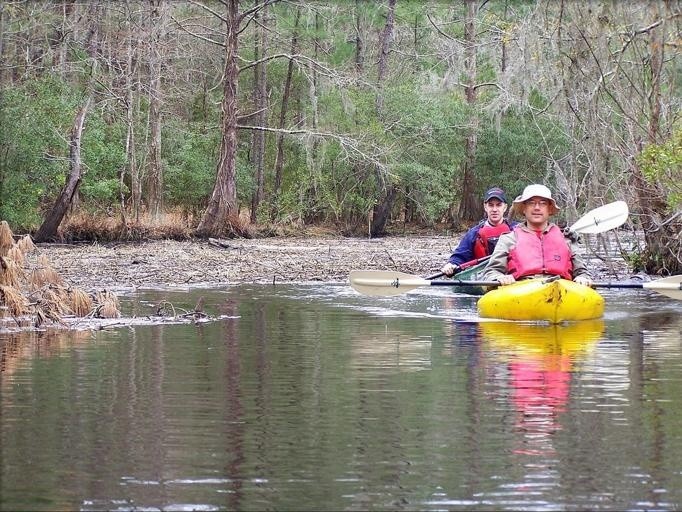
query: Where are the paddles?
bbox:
[350,269,681,298]
[425,201,628,278]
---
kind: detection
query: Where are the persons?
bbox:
[479,183,594,293]
[504,353,573,454]
[439,187,521,278]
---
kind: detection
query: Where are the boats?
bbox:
[452,261,502,296]
[477,276,606,325]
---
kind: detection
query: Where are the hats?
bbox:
[512,184,560,215]
[484,188,506,202]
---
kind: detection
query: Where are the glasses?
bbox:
[526,200,548,208]
